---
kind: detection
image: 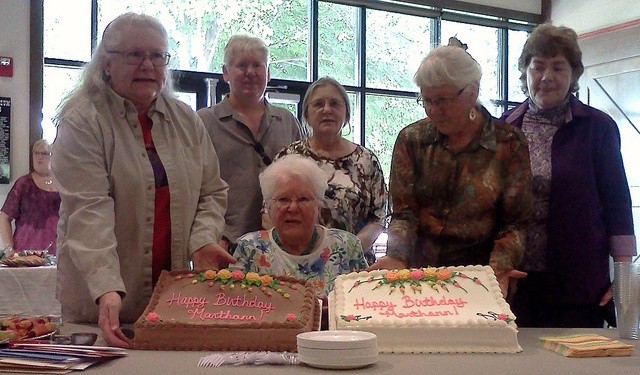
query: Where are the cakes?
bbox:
[333,264,519,346]
[130,268,320,353]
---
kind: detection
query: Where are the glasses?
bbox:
[106,50,171,67]
[254,140,271,166]
[308,99,347,108]
[416,83,470,108]
[272,192,317,207]
[32,150,52,157]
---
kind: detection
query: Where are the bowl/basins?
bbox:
[23,249,48,259]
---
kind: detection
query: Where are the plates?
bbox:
[296,329,379,370]
[24,329,58,341]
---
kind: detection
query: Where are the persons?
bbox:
[364,36,533,308]
[498,22,638,328]
[196,34,309,272]
[49,14,238,349]
[0,138,61,264]
[227,153,370,285]
[270,75,394,284]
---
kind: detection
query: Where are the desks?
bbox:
[1,262,61,318]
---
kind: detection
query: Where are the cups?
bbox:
[612,261,640,343]
[63,334,98,346]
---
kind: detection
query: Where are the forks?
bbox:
[197,349,300,368]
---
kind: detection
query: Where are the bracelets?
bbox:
[3,244,13,253]
